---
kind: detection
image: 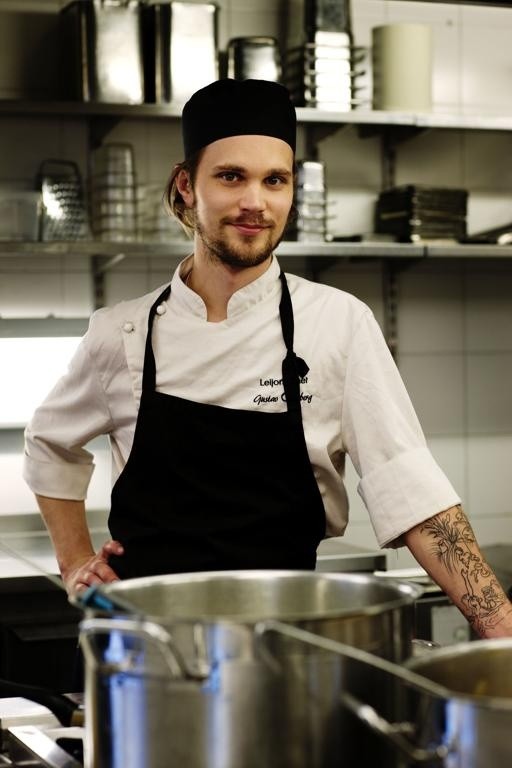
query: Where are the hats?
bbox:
[181,79,297,160]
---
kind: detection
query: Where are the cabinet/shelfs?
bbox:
[1,90,512,260]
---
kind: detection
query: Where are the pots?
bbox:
[338,637,512,768]
[83,569,415,768]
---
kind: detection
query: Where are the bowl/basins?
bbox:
[290,161,336,242]
[85,141,146,242]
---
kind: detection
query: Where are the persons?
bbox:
[22,78,512,643]
